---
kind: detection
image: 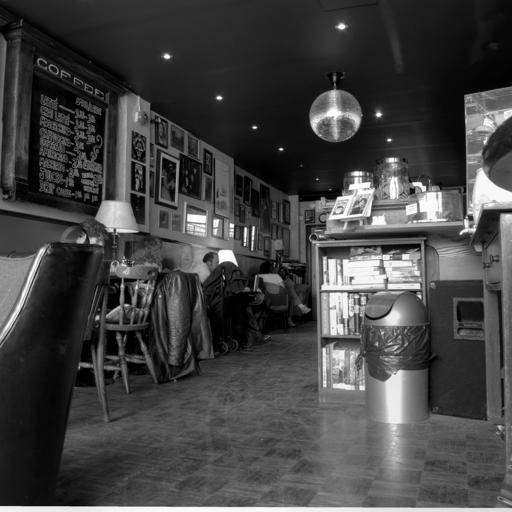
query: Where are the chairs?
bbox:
[98,264,160,394]
[0,241,104,505]
[81,259,113,423]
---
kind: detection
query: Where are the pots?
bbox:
[405,189,464,223]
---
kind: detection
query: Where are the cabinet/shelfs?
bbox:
[308,222,483,406]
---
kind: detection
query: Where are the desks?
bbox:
[470,201,512,465]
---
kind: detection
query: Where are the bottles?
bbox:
[377,157,410,199]
[343,170,374,195]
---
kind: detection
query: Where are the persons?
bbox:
[203,251,219,274]
[161,256,180,275]
[257,261,312,327]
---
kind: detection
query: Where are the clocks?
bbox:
[319,213,326,222]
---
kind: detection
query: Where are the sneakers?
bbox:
[288,322,295,327]
[295,306,310,315]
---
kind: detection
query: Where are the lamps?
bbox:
[269,239,284,270]
[309,71,364,142]
[94,200,139,263]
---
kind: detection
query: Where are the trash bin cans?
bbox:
[362,289,432,424]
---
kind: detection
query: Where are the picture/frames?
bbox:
[304,209,315,224]
[150,114,292,257]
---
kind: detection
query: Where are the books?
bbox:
[329,187,375,222]
[320,249,423,391]
